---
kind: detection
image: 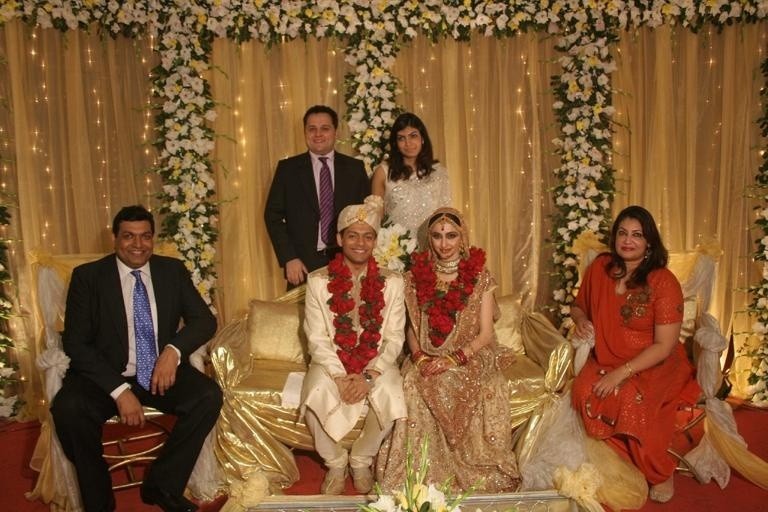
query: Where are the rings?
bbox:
[436,360,444,368]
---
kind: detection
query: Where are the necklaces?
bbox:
[431,256,466,274]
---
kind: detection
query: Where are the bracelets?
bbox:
[409,350,431,366]
[445,346,471,366]
[623,356,638,379]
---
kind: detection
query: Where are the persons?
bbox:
[303,204,402,493]
[568,203,704,502]
[401,207,495,487]
[264,105,370,286]
[370,112,452,248]
[49,206,223,510]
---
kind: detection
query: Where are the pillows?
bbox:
[242,297,309,364]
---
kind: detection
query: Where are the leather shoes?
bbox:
[141,483,198,512]
[650,474,674,503]
[349,465,372,493]
[321,466,348,495]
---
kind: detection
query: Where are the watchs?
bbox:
[364,370,375,387]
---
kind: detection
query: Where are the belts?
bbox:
[318,250,328,255]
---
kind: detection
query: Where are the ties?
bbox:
[131,271,158,391]
[318,158,335,246]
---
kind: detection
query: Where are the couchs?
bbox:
[214,283,575,488]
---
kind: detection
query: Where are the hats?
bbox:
[337,194,384,236]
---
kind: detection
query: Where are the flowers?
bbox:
[326,252,386,375]
[371,222,419,278]
[410,244,487,347]
[353,429,519,512]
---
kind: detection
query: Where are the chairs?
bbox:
[27,251,226,512]
[556,235,735,485]
